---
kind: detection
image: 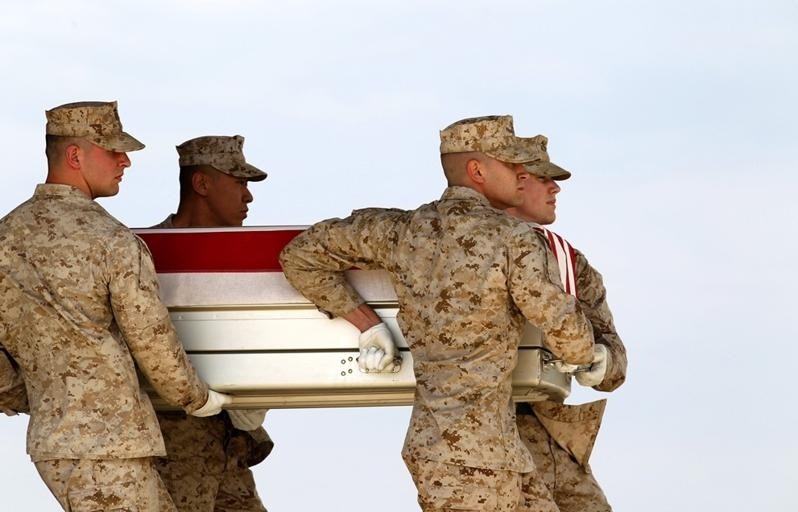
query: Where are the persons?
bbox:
[277,113,596,512]
[510,134,626,512]
[1,101,235,512]
[128,136,269,512]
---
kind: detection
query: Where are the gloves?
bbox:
[227,409,267,431]
[553,344,609,388]
[358,321,400,372]
[190,390,232,418]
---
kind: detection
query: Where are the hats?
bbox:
[439,115,541,165]
[516,134,571,181]
[175,134,268,182]
[43,101,146,154]
